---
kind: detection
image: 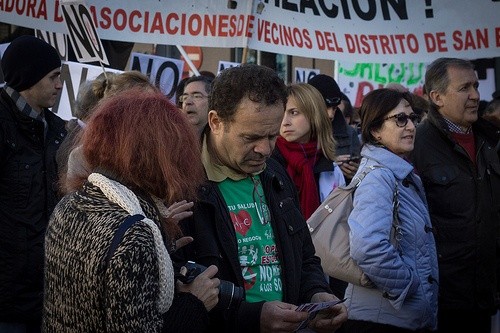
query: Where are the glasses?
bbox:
[384,113,418,127]
[252,176,270,226]
[179,94,211,102]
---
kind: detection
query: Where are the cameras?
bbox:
[177,260,246,317]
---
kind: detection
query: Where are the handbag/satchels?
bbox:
[306,165,403,288]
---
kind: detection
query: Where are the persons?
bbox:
[418,82,500,124]
[0,35,70,333]
[168,62,352,333]
[408,55,500,333]
[180,75,216,151]
[270,82,350,304]
[41,85,222,333]
[94,69,118,79]
[335,88,367,136]
[67,69,160,197]
[306,74,363,187]
[340,87,441,333]
[192,70,218,84]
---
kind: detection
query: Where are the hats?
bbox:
[307,74,342,106]
[0,34,62,92]
